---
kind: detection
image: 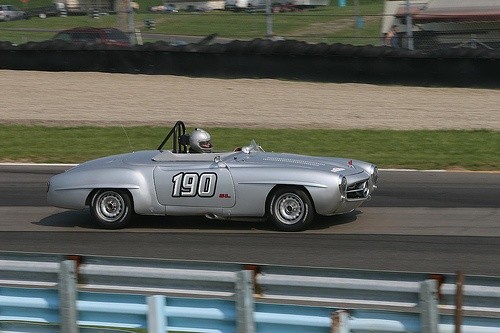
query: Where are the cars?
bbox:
[22,7,59,19]
[45,121,380,231]
[51,26,131,46]
[0,4,24,22]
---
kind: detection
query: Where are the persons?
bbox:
[385,24,399,47]
[188,130,213,151]
